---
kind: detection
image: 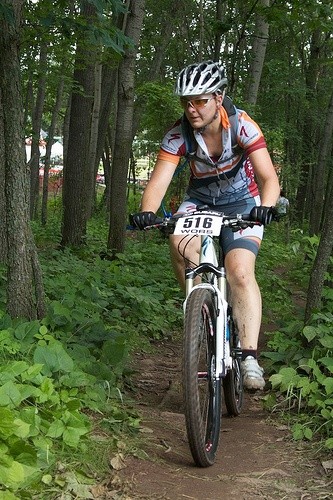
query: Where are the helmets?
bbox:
[175,61,228,95]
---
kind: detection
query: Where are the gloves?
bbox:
[250,206,274,225]
[132,212,158,231]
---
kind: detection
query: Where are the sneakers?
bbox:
[240,356,266,388]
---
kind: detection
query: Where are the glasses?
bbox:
[180,95,215,108]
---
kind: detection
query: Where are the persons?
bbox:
[133,62,281,390]
[275,191,289,214]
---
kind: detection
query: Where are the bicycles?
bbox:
[124,203,289,467]
